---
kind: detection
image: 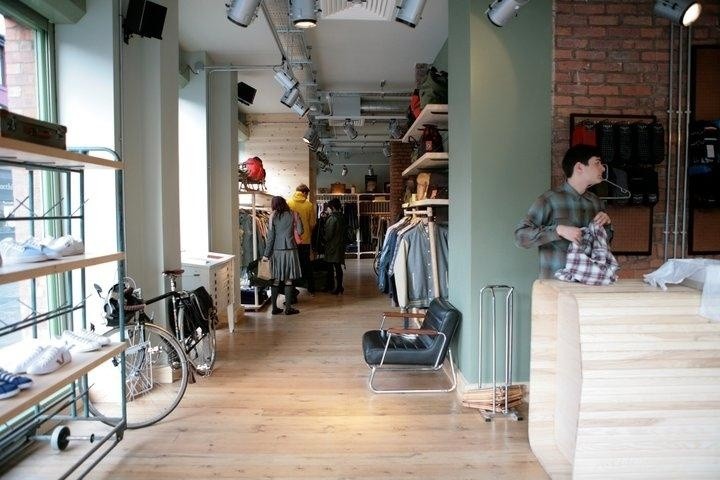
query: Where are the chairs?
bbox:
[361,295,462,393]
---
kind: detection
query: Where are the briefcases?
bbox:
[1,109,67,149]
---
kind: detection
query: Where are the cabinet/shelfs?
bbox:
[182,250,236,332]
[401,103,449,208]
[0,137,128,480]
[236,190,275,313]
[316,192,391,259]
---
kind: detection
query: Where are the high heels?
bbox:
[321,285,335,292]
[333,287,344,295]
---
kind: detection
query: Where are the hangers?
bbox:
[405,210,428,227]
[592,163,631,201]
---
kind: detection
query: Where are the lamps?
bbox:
[653,0,703,27]
[273,61,400,176]
[487,0,529,27]
[290,0,317,28]
[396,0,427,29]
[226,0,262,28]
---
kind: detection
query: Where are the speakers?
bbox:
[124,0,168,39]
[238,82,257,105]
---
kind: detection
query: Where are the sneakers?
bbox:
[273,308,284,314]
[0,234,83,263]
[285,309,299,314]
[0,327,112,398]
[308,286,319,292]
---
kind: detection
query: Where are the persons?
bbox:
[287,184,317,297]
[264,196,300,315]
[320,198,348,295]
[513,144,613,278]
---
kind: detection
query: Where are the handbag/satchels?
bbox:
[409,125,443,164]
[257,259,272,281]
[404,171,448,201]
[406,65,448,126]
[169,285,218,339]
[238,157,266,182]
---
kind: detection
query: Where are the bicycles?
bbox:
[76,267,219,433]
